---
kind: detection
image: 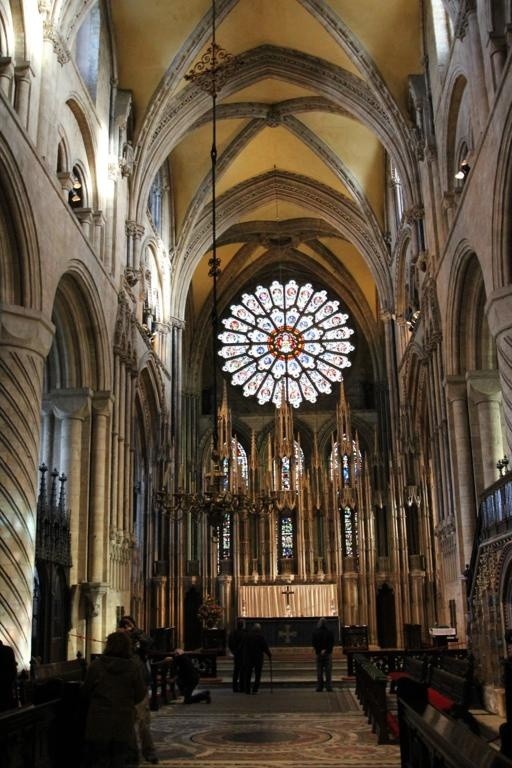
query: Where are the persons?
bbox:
[106,616,149,698]
[228,619,272,694]
[311,618,334,692]
[165,648,211,704]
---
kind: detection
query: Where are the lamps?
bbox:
[152,0,281,543]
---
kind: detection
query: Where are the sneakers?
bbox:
[205,691,212,704]
[316,687,334,692]
[233,687,260,695]
